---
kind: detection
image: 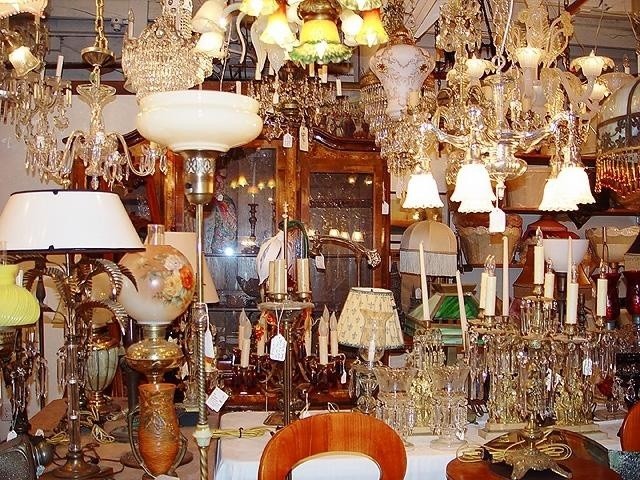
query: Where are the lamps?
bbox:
[3,1,639,478]
